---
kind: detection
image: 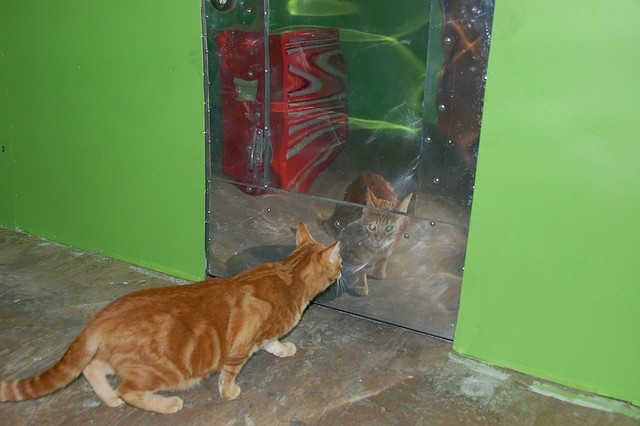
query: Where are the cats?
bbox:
[314,169,414,296]
[0,221,345,416]
